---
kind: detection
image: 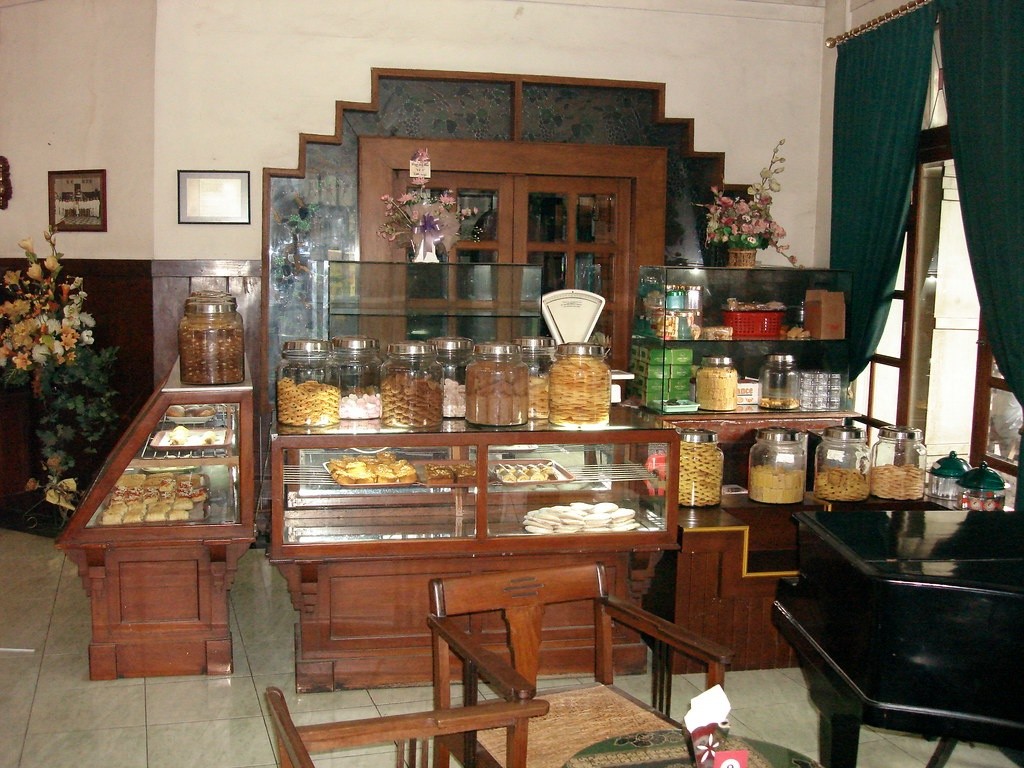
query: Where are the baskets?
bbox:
[722,308,785,336]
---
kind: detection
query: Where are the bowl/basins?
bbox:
[929,452,972,499]
[956,461,1010,511]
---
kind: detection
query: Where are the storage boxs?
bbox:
[628,344,693,408]
[737,379,763,404]
[804,290,848,339]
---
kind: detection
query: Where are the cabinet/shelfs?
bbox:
[629,265,862,455]
[270,411,682,695]
[55,350,256,681]
[328,261,543,346]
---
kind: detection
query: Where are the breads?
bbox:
[691,325,734,340]
[101,473,208,524]
[164,405,215,445]
[326,451,479,485]
[493,462,559,483]
[781,324,810,338]
[523,502,639,534]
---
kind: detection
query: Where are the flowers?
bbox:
[689,138,806,268]
[376,147,483,260]
[0,225,119,523]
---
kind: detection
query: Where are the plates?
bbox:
[653,400,700,412]
[167,415,213,424]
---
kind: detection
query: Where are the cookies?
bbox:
[749,464,924,504]
[760,398,801,409]
[379,356,611,430]
[277,377,342,428]
[679,443,723,509]
[696,367,738,412]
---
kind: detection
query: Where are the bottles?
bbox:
[547,255,602,294]
[748,429,807,504]
[381,342,443,429]
[466,343,528,426]
[638,276,703,340]
[276,340,340,427]
[678,428,724,506]
[758,354,802,409]
[872,426,927,500]
[549,342,611,428]
[511,336,557,419]
[331,336,382,419]
[426,338,474,417]
[178,290,245,384]
[814,426,871,501]
[696,357,738,411]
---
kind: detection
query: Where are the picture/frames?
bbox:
[177,169,250,225]
[48,169,107,232]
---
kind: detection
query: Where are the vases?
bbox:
[411,239,440,263]
[728,250,757,267]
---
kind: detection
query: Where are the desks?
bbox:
[562,728,824,768]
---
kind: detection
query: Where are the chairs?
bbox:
[266,687,549,768]
[426,561,734,768]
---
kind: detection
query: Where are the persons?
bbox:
[55,197,100,219]
